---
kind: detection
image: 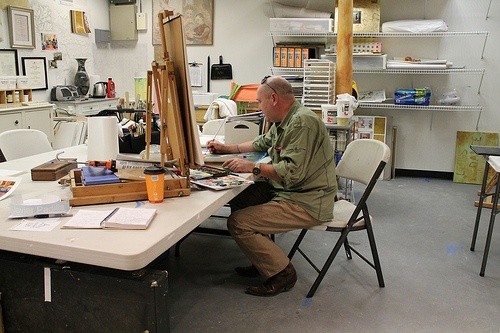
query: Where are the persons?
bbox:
[205,76,338,297]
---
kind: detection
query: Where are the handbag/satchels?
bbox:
[229,180,275,214]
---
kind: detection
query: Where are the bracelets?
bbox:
[237,145,240,152]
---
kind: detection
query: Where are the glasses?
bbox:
[261,75,278,95]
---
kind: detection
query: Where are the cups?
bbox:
[144,166,165,202]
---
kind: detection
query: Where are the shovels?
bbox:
[211,56,232,80]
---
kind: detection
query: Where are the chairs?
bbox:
[286,139,391,298]
[0,129,53,162]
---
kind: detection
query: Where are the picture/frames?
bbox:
[152,0,214,45]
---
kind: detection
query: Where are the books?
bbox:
[60,208,158,230]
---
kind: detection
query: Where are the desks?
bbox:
[470,145,500,277]
[0,135,275,333]
[324,119,356,203]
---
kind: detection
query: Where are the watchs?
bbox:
[253,163,261,175]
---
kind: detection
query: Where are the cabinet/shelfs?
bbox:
[0,102,53,146]
[49,97,119,117]
[264,0,492,130]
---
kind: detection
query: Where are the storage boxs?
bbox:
[270,17,332,33]
[202,119,260,146]
[321,53,388,70]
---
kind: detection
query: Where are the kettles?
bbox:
[93,82,107,98]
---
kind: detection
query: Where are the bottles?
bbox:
[138,100,146,109]
[139,119,146,135]
[107,78,115,98]
[117,104,135,120]
[74,58,90,98]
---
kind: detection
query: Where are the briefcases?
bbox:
[43,262,171,333]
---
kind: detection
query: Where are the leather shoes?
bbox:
[246,277,297,296]
[234,264,262,280]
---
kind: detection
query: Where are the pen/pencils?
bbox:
[35,214,73,218]
[204,117,228,155]
